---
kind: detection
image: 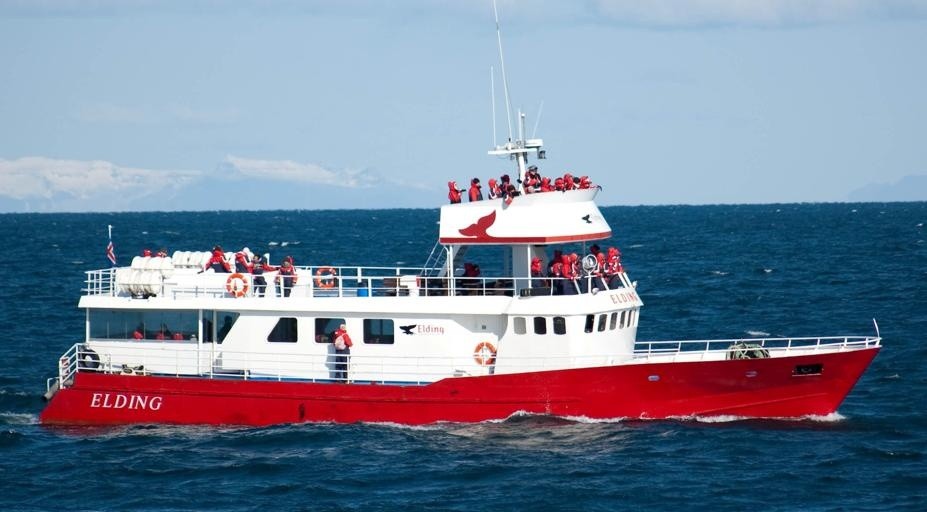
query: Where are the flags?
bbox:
[106,237,116,267]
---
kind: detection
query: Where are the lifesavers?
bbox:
[472,341,495,365]
[227,274,249,297]
[314,268,338,289]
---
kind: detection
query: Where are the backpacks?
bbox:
[334,333,347,351]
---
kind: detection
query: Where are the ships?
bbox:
[39,2,884,429]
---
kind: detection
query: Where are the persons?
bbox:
[134,315,234,344]
[332,324,353,385]
[196,244,297,297]
[446,165,625,293]
